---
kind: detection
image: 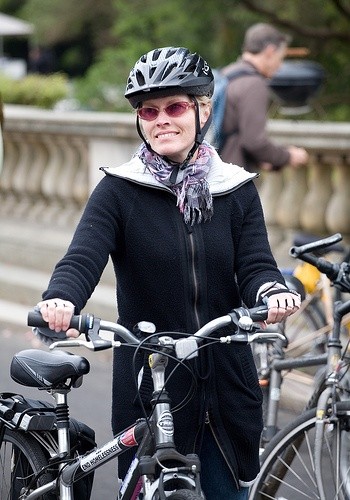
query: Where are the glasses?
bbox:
[133,100,195,120]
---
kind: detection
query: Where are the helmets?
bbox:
[124,45,216,105]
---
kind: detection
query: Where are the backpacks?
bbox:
[206,67,261,152]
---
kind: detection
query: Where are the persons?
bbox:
[31,45,301,500]
[209,23,309,176]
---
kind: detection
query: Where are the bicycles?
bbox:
[1,303,267,500]
[250,235,350,500]
